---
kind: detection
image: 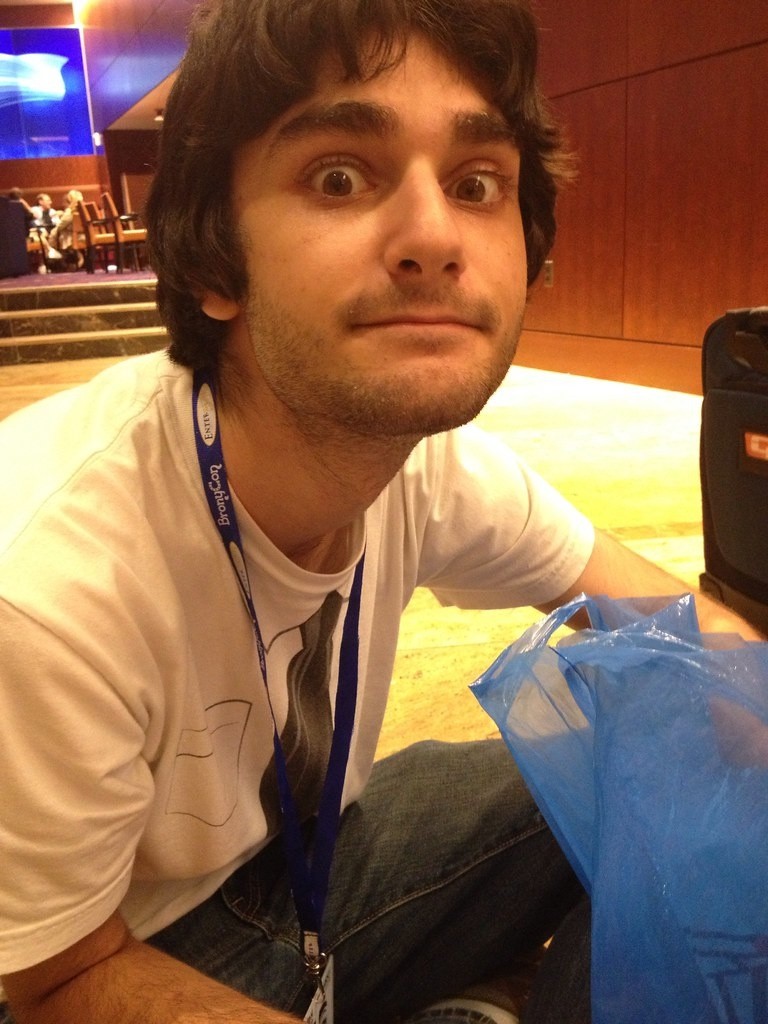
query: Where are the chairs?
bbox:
[0,191,147,275]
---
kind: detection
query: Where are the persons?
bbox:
[0,0,768,1024]
[6,188,85,274]
